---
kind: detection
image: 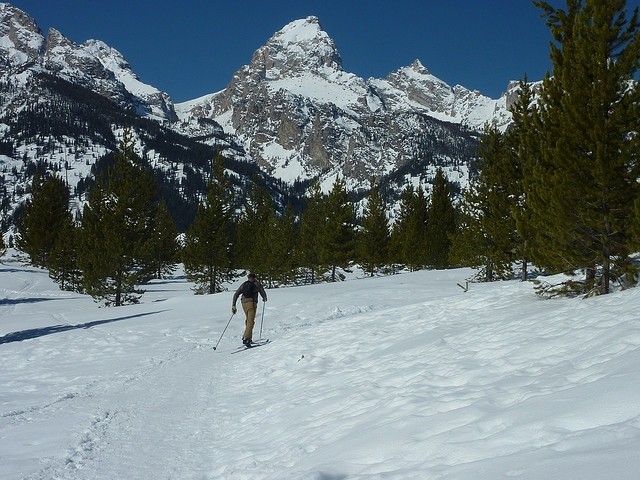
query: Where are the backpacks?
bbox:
[242,281,257,298]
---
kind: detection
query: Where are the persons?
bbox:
[231,273,268,347]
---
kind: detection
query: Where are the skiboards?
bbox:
[231,338,272,354]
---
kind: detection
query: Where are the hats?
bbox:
[247,273,257,278]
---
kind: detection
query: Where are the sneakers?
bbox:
[243,338,251,347]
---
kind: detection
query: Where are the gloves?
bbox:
[263,296,267,302]
[232,305,237,315]
